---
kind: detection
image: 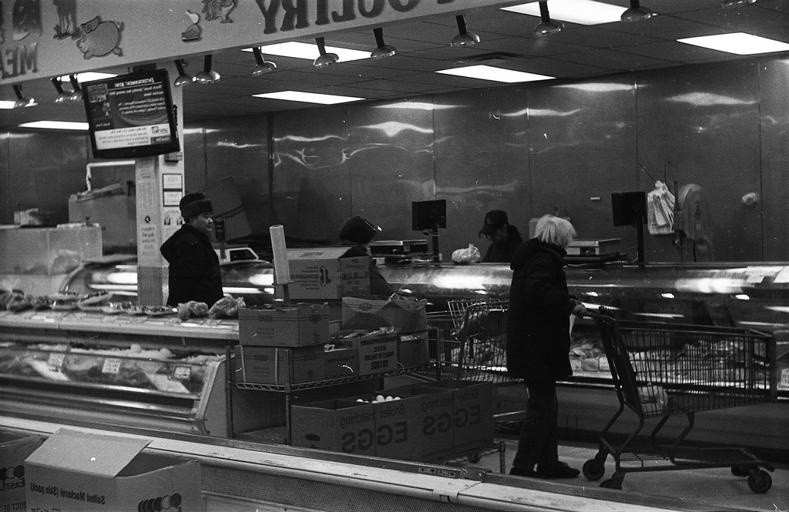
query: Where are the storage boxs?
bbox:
[232,256,498,464]
[1,426,31,511]
[23,425,202,509]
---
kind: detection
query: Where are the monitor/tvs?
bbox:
[612,191,647,224]
[412,198,446,230]
[81,69,180,159]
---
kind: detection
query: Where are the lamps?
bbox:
[12,0,662,116]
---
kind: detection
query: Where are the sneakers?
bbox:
[509,461,582,479]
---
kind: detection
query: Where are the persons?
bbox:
[336,216,398,298]
[508,214,587,479]
[160,193,225,311]
[480,210,523,262]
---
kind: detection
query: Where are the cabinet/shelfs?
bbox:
[0,303,292,438]
[89,257,277,303]
[383,256,789,465]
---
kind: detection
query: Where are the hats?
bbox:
[179,191,214,221]
[479,209,509,236]
